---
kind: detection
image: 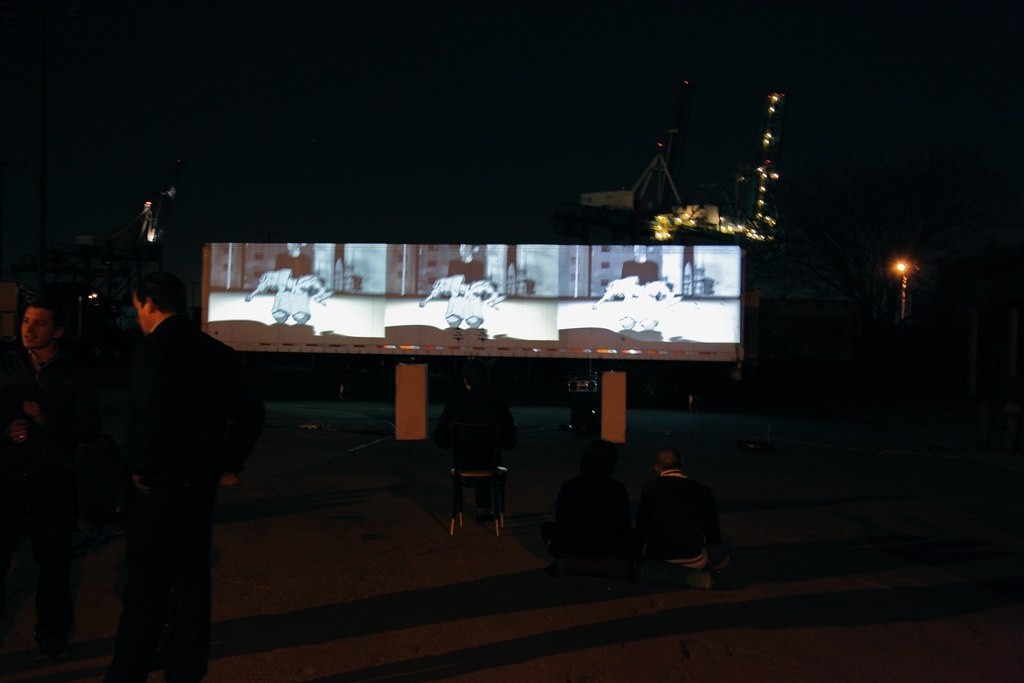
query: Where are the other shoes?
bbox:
[35,629,79,662]
[477,512,492,520]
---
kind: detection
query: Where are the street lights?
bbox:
[888,257,918,321]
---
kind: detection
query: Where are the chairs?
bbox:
[447,420,508,536]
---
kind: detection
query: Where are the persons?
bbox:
[542,440,634,562]
[431,365,518,497]
[0,298,99,662]
[103,273,265,683]
[636,446,731,584]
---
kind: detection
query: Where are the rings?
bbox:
[19,435,24,439]
